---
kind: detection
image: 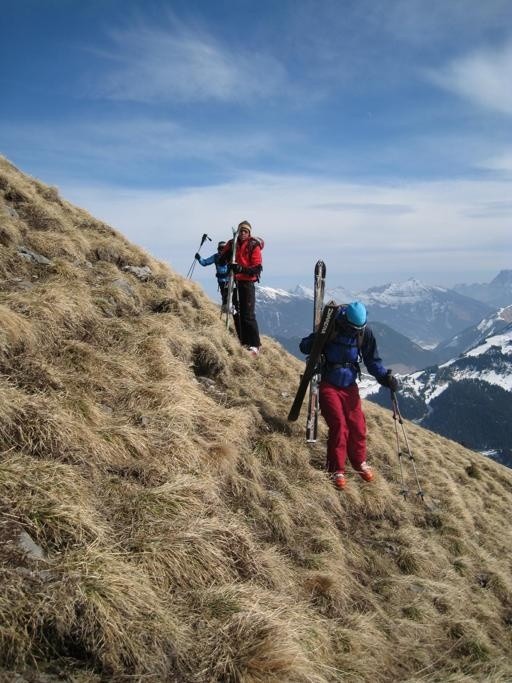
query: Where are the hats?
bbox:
[238,221,251,232]
[345,303,367,329]
[219,240,226,247]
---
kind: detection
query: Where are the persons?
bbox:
[195,220,263,357]
[299,302,401,490]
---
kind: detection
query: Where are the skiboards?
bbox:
[222,225,242,330]
[287,258,340,444]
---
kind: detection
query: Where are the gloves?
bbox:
[195,253,200,260]
[229,264,242,273]
[384,368,398,392]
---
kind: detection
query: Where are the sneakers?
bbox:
[351,462,373,483]
[331,472,345,490]
[248,345,259,358]
